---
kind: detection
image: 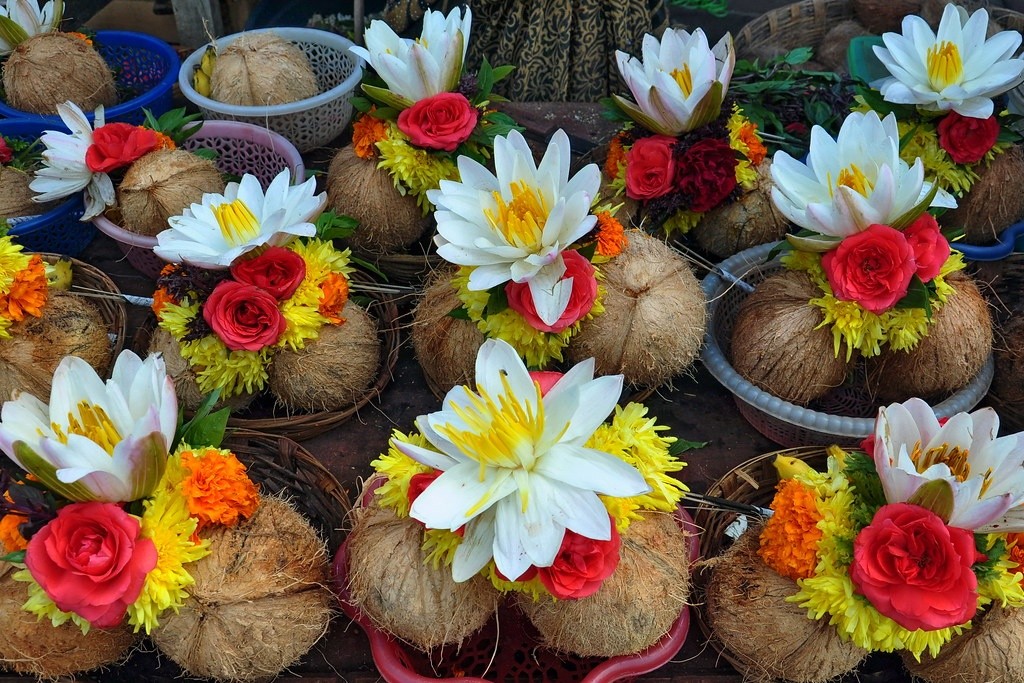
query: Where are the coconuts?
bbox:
[0,1,1024,683]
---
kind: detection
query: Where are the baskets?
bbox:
[690,447,908,683]
[732,1,1024,66]
[346,239,451,298]
[1,28,366,281]
[22,252,126,383]
[114,428,353,683]
[698,241,994,447]
[133,269,400,441]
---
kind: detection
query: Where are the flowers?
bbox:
[427,127,624,366]
[0,0,68,60]
[1,352,259,636]
[345,7,524,207]
[145,169,351,398]
[26,100,177,222]
[367,338,688,596]
[1,235,70,343]
[760,396,1024,660]
[608,28,767,210]
[771,4,1024,365]
